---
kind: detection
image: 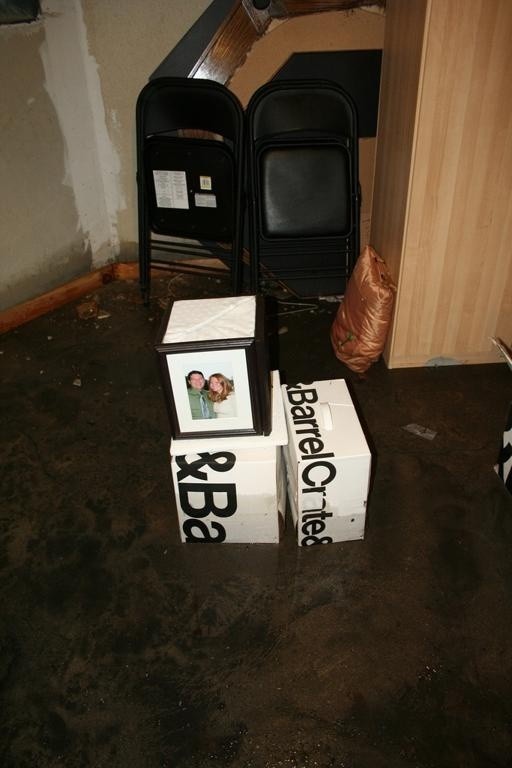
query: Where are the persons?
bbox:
[186,371,217,419]
[206,374,237,418]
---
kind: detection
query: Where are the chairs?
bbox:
[135,76,362,322]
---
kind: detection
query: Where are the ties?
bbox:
[201,396,208,417]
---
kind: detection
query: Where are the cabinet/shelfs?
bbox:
[370,0,512,372]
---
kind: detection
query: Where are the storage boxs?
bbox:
[168,372,281,547]
[154,292,272,438]
[283,376,372,547]
